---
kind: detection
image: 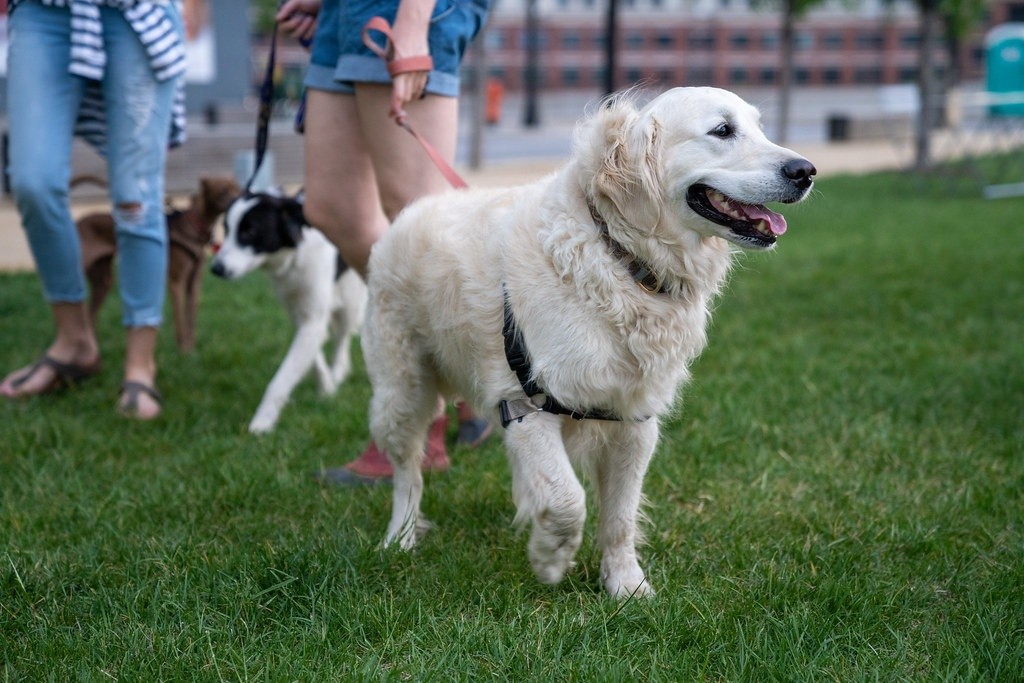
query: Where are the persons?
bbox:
[275,0,493,486]
[0,0,204,418]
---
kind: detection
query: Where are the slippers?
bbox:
[2,337,104,401]
[112,363,161,425]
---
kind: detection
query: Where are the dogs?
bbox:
[211,188,368,436]
[68,175,242,355]
[364,86,817,595]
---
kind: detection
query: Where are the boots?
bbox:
[452,396,491,454]
[323,413,448,492]
